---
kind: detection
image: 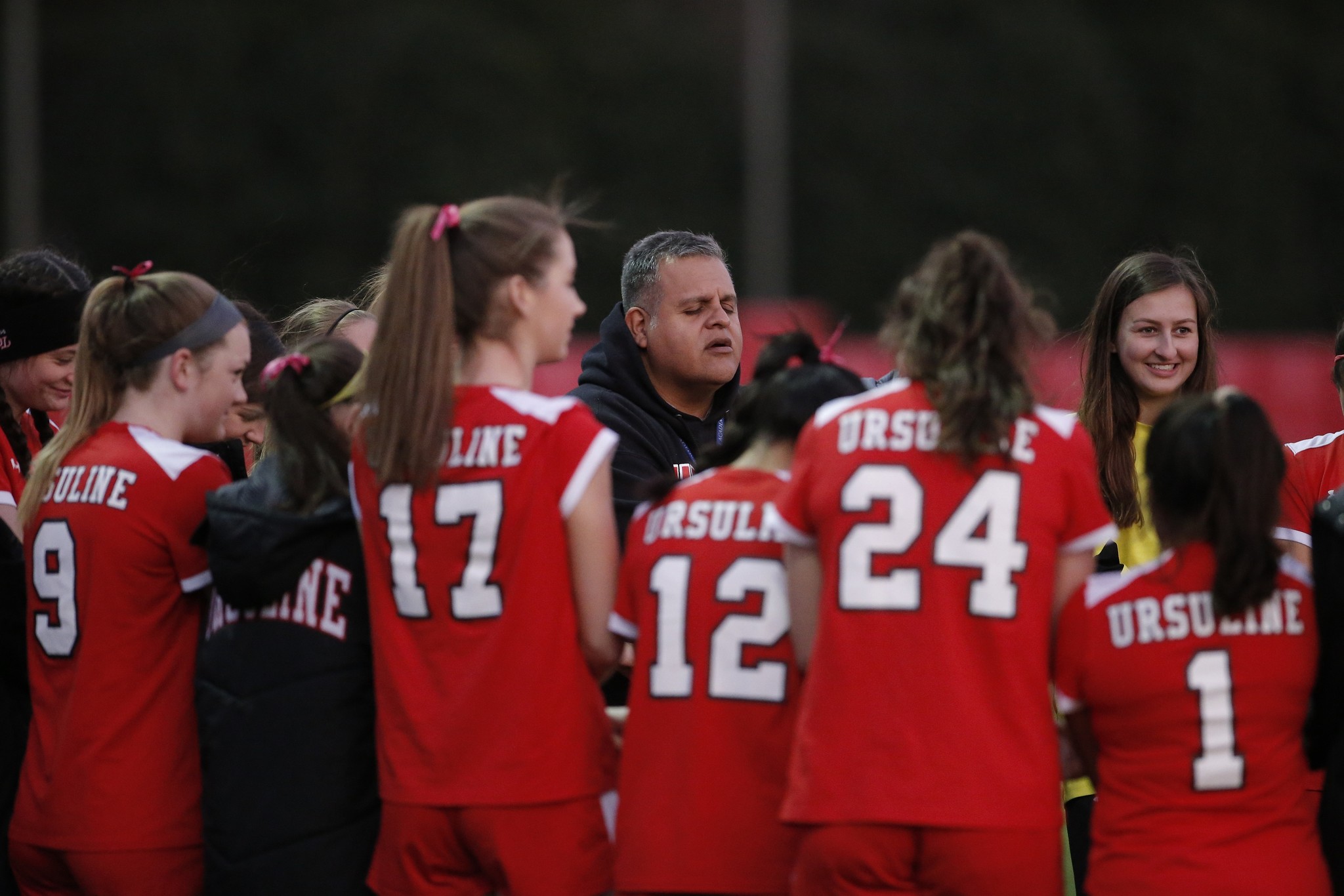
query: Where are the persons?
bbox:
[342,199,633,894]
[609,329,863,896]
[767,227,1118,895]
[1056,253,1218,896]
[569,230,758,714]
[1054,389,1333,895]
[0,251,389,896]
[1273,331,1344,575]
